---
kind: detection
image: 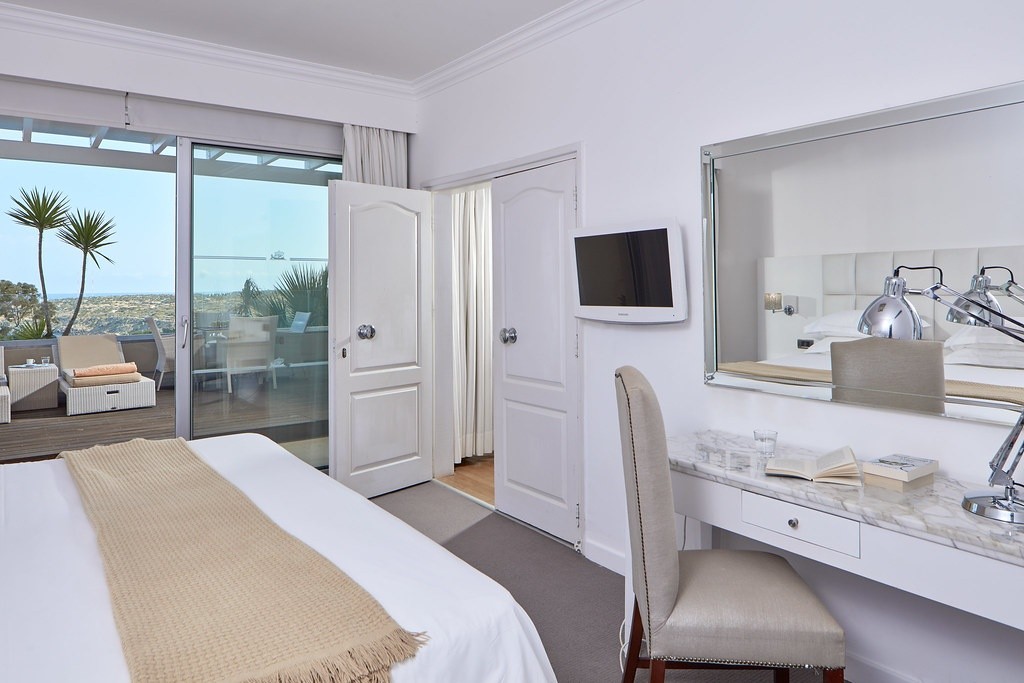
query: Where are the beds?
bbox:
[716,245,1024,414]
[0,432,558,683]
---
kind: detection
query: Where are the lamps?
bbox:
[946,266,1024,328]
[857,266,1024,524]
[764,291,799,316]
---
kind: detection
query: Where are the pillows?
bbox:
[804,309,931,339]
[944,349,1024,370]
[803,337,861,355]
[943,316,1024,351]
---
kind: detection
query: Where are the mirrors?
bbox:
[700,80,1024,428]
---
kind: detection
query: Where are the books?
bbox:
[863,472,935,492]
[863,453,939,482]
[764,446,862,487]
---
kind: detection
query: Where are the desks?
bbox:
[7,363,59,412]
[207,340,228,362]
[625,424,1024,683]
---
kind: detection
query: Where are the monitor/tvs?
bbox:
[569,219,687,323]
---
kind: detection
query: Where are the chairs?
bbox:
[145,316,206,392]
[51,335,156,416]
[216,314,278,394]
[0,346,11,424]
[614,365,845,683]
[277,311,311,381]
